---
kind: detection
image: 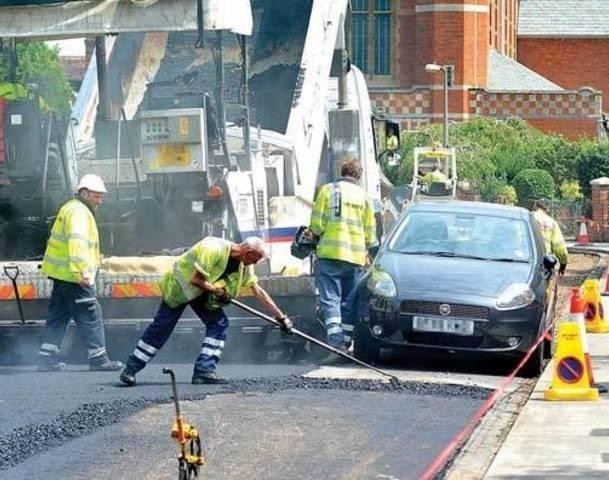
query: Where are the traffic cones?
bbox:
[569,285,609,394]
[580,276,609,334]
[600,273,609,296]
[574,214,595,245]
[540,320,600,402]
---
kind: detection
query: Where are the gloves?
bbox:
[216,287,232,305]
[276,315,293,335]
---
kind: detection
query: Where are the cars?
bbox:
[348,198,563,376]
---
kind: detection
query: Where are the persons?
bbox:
[119,236,294,385]
[418,163,450,196]
[309,159,377,365]
[386,127,399,151]
[36,173,124,372]
[530,198,568,280]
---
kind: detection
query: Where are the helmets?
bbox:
[291,225,318,259]
[76,174,108,195]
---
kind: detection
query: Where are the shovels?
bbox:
[4,266,37,324]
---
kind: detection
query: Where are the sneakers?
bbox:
[193,373,227,384]
[89,359,124,371]
[119,368,136,384]
[317,345,350,366]
[37,361,67,371]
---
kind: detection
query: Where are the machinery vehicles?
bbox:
[0,1,403,366]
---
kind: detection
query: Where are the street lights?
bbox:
[423,62,450,148]
